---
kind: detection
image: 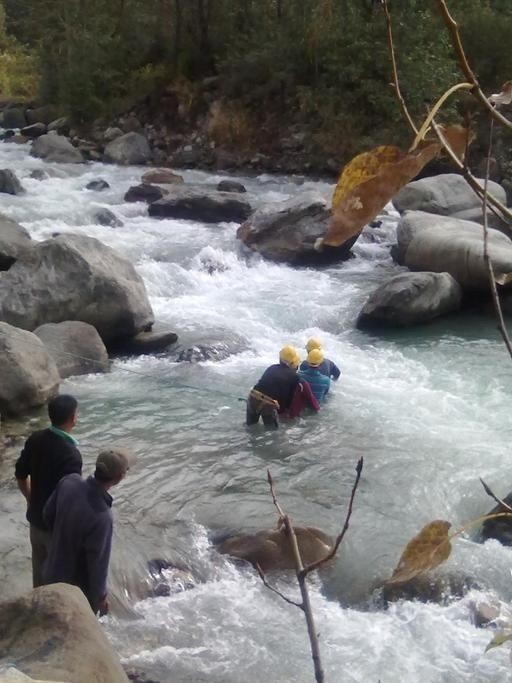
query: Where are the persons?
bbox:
[14,394,84,589]
[39,447,131,615]
[277,352,321,424]
[246,346,299,430]
[299,338,341,382]
[296,347,330,409]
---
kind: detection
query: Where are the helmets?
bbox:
[280,346,296,362]
[307,339,321,351]
[308,348,323,366]
[287,353,301,370]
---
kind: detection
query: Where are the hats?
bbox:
[95,445,137,479]
